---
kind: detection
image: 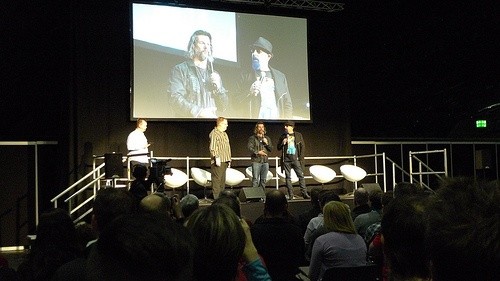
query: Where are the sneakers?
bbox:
[304,195,312,199]
[289,196,294,200]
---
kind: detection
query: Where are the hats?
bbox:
[248,37,274,58]
[282,121,296,128]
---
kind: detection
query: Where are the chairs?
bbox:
[246,167,272,184]
[321,264,378,281]
[340,165,367,198]
[276,166,300,200]
[191,167,211,204]
[164,167,187,195]
[226,168,245,189]
[309,165,336,188]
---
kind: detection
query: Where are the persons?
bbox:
[208,117,231,199]
[277,121,311,200]
[168,30,229,118]
[230,36,293,119]
[0,176,500,281]
[248,124,273,190]
[127,118,150,165]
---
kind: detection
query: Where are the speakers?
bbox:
[238,187,265,202]
[104,152,124,179]
[360,183,381,193]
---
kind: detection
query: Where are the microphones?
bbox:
[284,133,289,145]
[262,132,264,143]
[207,56,215,87]
[254,71,266,96]
